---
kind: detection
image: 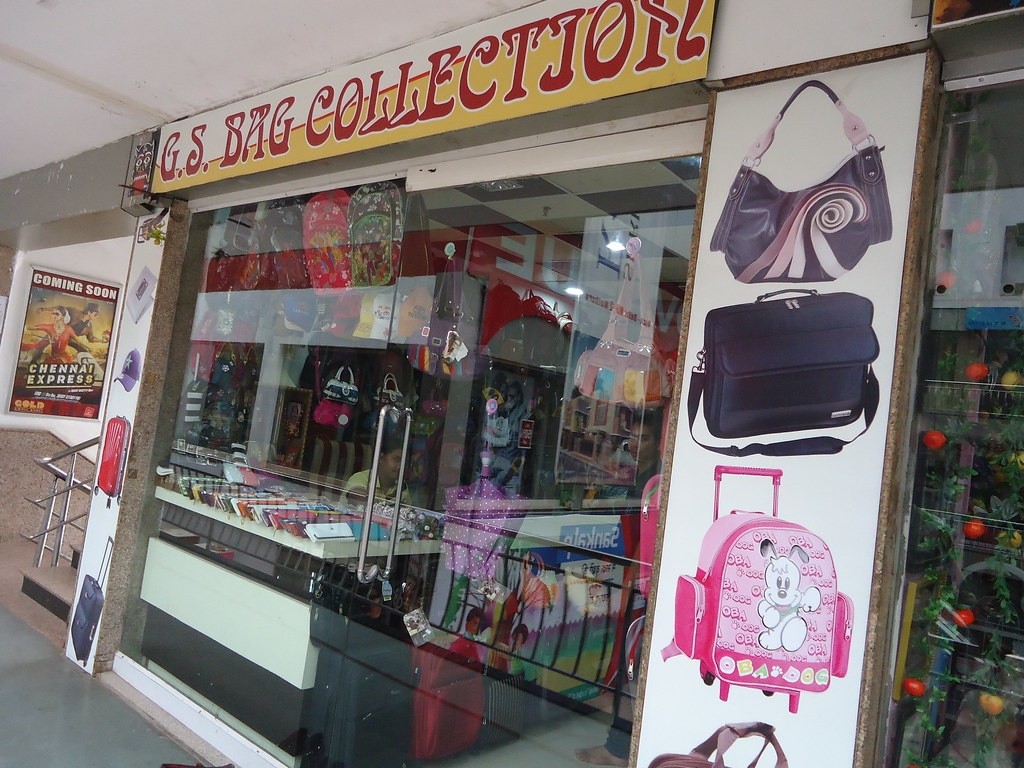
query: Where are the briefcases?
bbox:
[647,721,789,768]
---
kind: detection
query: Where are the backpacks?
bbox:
[640,474,661,599]
[302,190,352,288]
[243,195,308,289]
[346,181,432,286]
[624,616,645,712]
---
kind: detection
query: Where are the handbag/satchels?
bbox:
[323,366,358,405]
[407,256,492,380]
[410,416,436,439]
[308,557,352,642]
[314,400,352,428]
[710,79,893,284]
[686,289,881,457]
[422,389,447,416]
[175,342,259,446]
[575,253,676,410]
[361,409,398,434]
[374,373,404,408]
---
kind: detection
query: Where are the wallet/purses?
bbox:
[126,266,157,324]
[178,463,391,543]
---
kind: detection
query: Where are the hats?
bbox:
[114,347,141,391]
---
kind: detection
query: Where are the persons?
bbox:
[27,306,94,371]
[337,436,412,511]
[29,302,109,366]
[575,412,663,768]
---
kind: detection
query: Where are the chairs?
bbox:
[306,437,374,503]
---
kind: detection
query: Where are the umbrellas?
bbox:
[442,448,534,581]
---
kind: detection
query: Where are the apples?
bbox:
[1000,368,1021,390]
[998,530,1021,548]
[980,693,1003,715]
[991,450,1024,483]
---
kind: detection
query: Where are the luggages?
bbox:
[300,643,412,768]
[71,537,114,667]
[662,465,854,714]
[94,415,131,509]
[408,632,483,768]
[484,646,527,745]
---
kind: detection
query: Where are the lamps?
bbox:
[605,232,626,252]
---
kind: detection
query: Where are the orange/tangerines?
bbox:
[964,362,989,382]
[903,676,924,696]
[923,430,945,450]
[952,608,974,627]
[964,519,986,539]
